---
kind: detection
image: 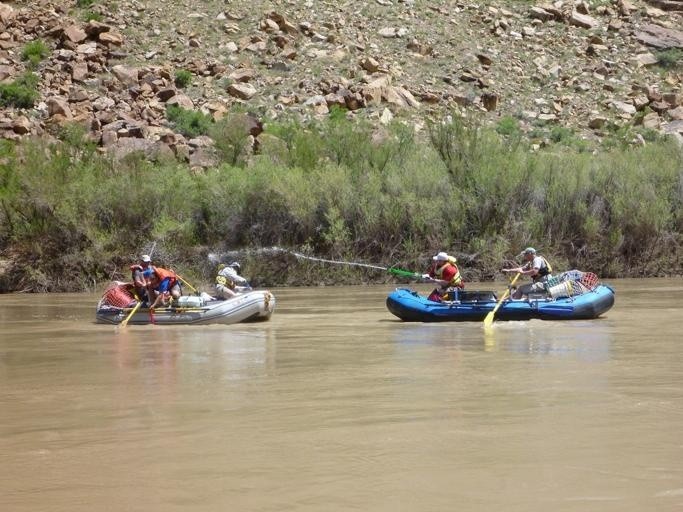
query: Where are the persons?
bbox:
[421,251,465,303]
[130,254,159,303]
[141,265,181,310]
[502,246,553,301]
[213,261,247,300]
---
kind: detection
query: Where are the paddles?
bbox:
[461,296,552,304]
[97,307,212,314]
[118,300,143,329]
[170,268,201,297]
[484,272,520,325]
[425,304,495,308]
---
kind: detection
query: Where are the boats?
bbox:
[96,290,276,324]
[386,283,614,320]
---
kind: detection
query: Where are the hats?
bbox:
[229,262,241,268]
[432,252,448,261]
[140,255,151,262]
[521,247,536,255]
